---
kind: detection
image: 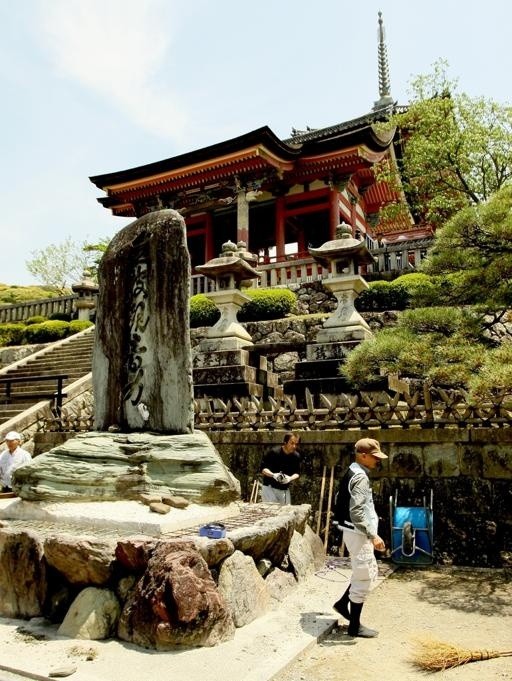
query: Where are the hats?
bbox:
[3,431,20,442]
[354,437,389,460]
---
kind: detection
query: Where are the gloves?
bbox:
[281,473,291,484]
[272,472,284,482]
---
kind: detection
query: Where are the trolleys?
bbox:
[383,487,438,570]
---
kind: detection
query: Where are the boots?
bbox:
[333,582,353,621]
[347,598,379,639]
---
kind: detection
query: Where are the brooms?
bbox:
[399,632,512,681]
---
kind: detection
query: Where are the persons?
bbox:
[260,433,302,505]
[0,431,33,493]
[332,438,389,637]
